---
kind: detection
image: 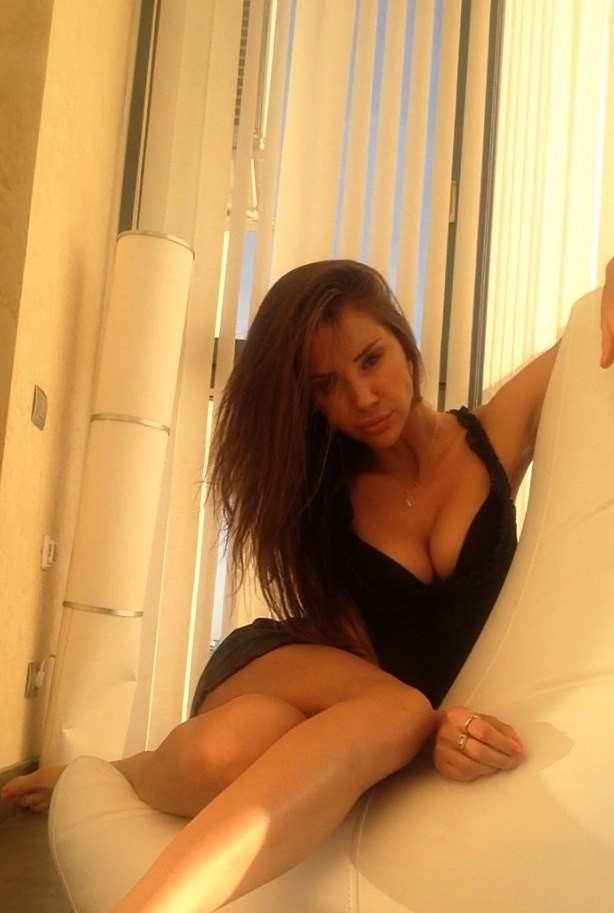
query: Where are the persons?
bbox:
[0,256,614,913]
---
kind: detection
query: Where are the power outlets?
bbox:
[25,661,42,698]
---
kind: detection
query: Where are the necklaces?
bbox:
[388,411,439,510]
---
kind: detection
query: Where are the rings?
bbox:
[456,734,470,751]
[464,713,478,733]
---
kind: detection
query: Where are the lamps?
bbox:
[42,231,205,765]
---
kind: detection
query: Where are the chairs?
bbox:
[46,291,614,913]
